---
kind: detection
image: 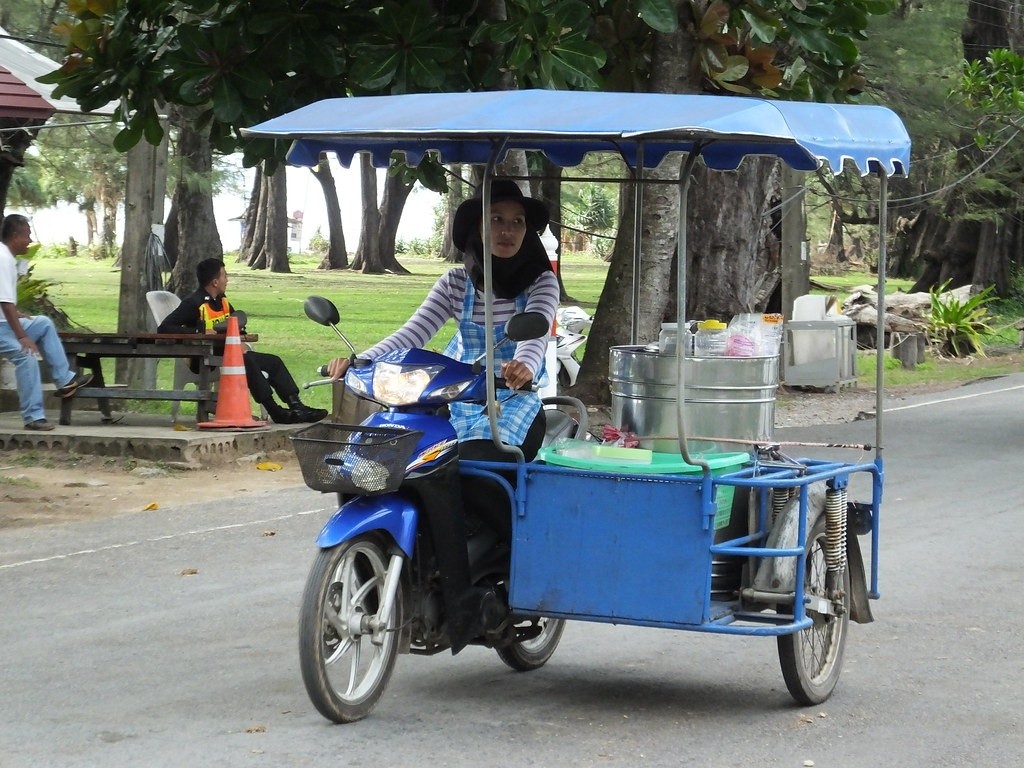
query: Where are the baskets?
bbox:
[289,423,426,499]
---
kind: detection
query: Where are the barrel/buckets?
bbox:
[609,344,783,602]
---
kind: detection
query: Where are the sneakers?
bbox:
[26,419,55,431]
[58,372,95,399]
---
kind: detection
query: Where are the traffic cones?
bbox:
[195,316,268,431]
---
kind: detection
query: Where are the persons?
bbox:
[156,258,328,423]
[330,181,560,462]
[0,214,94,430]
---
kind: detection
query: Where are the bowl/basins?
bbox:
[538,443,751,530]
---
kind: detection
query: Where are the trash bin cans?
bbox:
[787,291,857,392]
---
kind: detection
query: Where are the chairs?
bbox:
[146,290,269,422]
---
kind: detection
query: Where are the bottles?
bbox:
[658,322,693,356]
[694,320,729,357]
[324,451,390,491]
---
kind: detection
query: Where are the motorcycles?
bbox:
[236,88,910,724]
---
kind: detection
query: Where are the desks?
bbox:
[54,332,258,426]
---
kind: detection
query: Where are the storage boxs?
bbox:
[538,446,750,530]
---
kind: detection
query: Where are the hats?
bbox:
[453,179,549,252]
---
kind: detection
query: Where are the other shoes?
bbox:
[269,405,304,423]
[290,403,328,423]
